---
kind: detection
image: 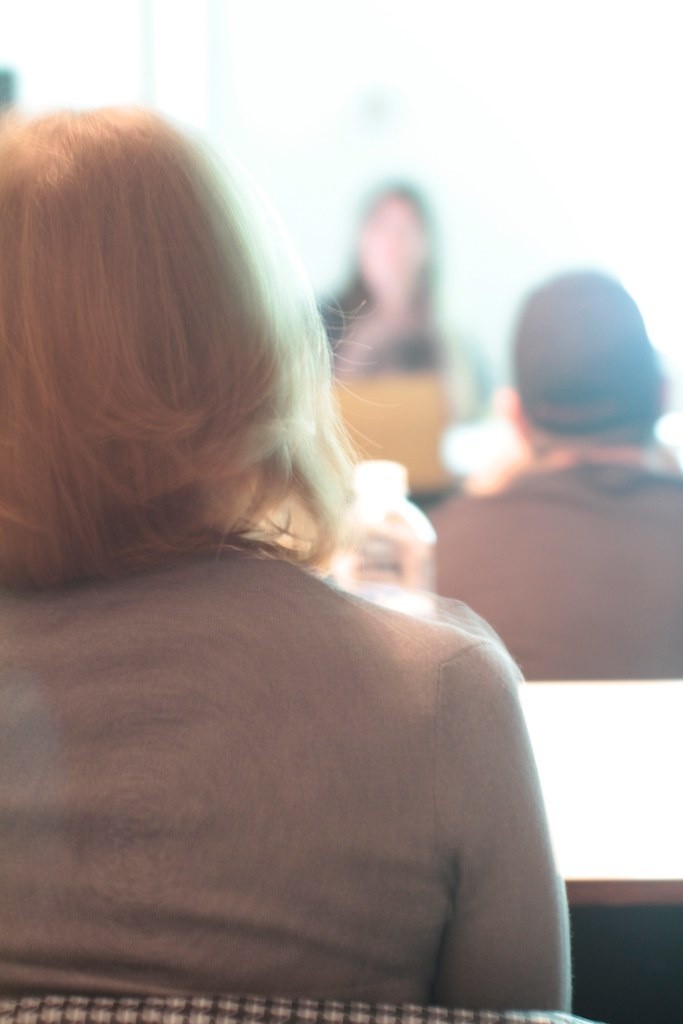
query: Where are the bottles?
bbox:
[337,459,433,618]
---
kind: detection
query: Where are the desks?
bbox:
[519,677,680,908]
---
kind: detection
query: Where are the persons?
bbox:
[0,105,572,1014]
[318,183,497,514]
[423,270,683,682]
